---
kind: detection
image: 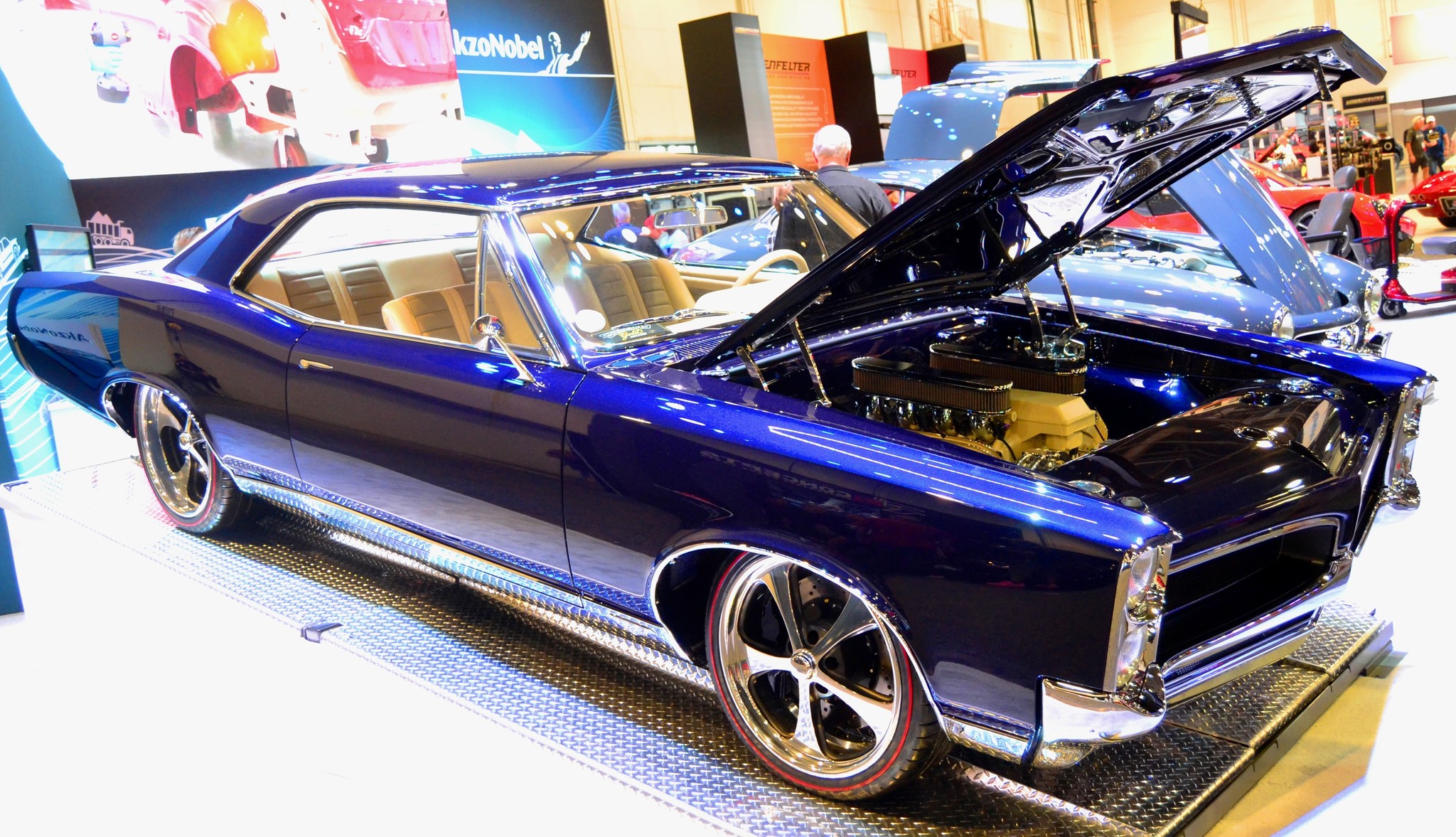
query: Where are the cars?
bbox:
[668,56,1395,367]
[6,21,1440,805]
[1109,152,1418,263]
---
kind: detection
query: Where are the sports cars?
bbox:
[1409,168,1456,227]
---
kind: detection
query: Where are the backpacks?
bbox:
[1403,128,1416,148]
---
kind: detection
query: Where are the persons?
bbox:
[1421,115,1451,178]
[1232,127,1396,178]
[1404,115,1430,192]
[604,202,643,250]
[633,214,668,259]
[657,224,691,261]
[766,123,897,272]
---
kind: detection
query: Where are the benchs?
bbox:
[268,234,586,349]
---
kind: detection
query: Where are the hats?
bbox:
[1425,115,1436,123]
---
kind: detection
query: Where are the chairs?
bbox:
[380,283,544,352]
[592,256,693,332]
